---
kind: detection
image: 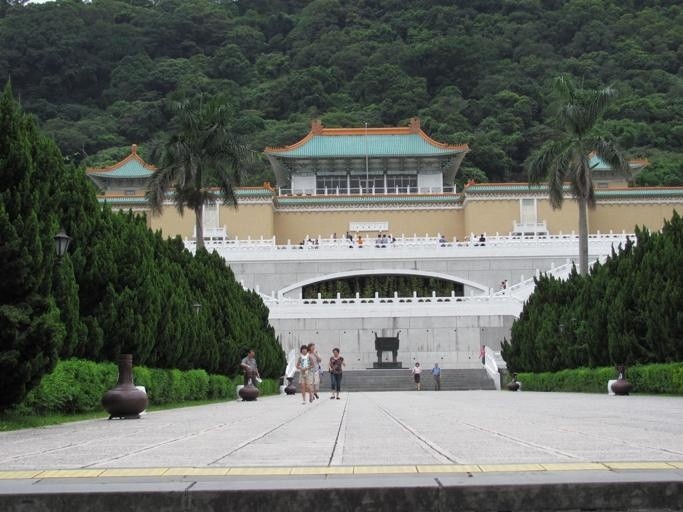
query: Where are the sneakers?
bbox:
[309,393,319,403]
[330,396,340,399]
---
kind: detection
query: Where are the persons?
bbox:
[409,362,422,391]
[503,279,508,295]
[294,345,314,404]
[240,349,260,388]
[500,282,504,296]
[306,342,322,399]
[298,231,398,251]
[440,231,488,247]
[326,347,346,400]
[478,344,486,365]
[429,364,441,391]
